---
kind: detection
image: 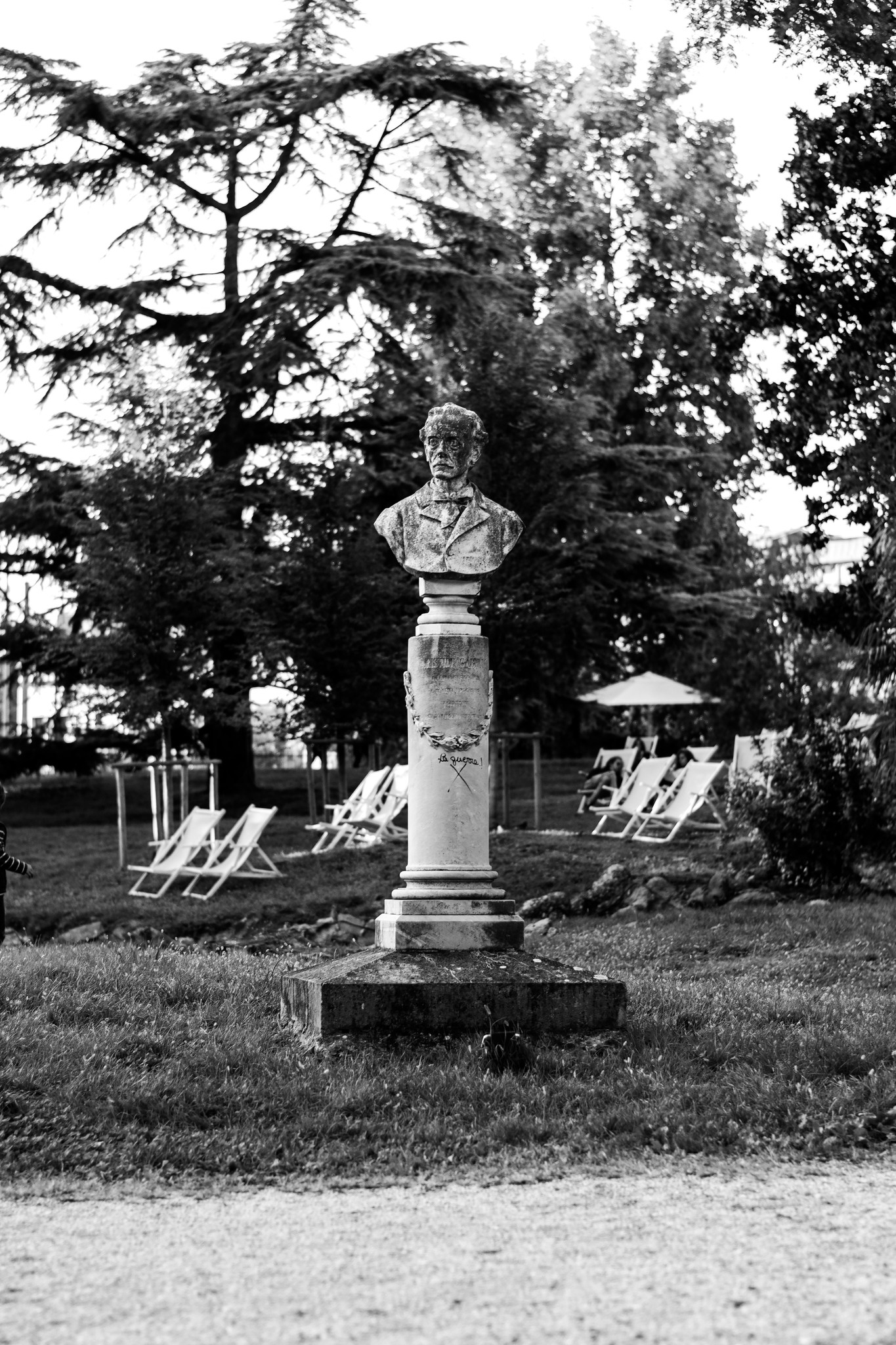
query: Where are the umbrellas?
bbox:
[576,670,726,737]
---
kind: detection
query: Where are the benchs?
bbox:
[125,806,226,901]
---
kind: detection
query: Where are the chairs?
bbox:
[340,762,408,849]
[726,730,782,811]
[624,734,660,759]
[302,763,391,853]
[576,745,640,814]
[182,802,288,899]
[589,750,680,836]
[635,761,730,845]
[665,743,723,803]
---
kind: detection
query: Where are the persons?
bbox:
[373,402,525,580]
[0,785,35,946]
[583,738,699,805]
